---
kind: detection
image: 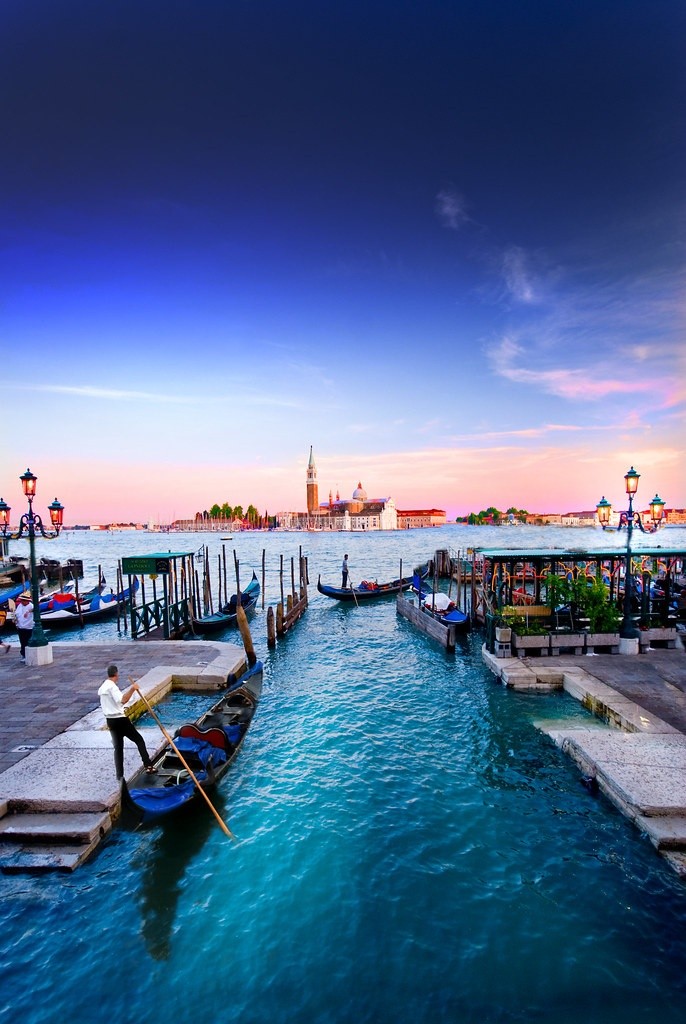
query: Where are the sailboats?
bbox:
[156,510,333,532]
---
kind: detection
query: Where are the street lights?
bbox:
[0,467,66,647]
[595,465,668,639]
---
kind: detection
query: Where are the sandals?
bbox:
[146,767,158,774]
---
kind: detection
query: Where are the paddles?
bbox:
[128,675,236,843]
[347,573,360,608]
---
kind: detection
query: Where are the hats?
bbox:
[18,593,32,601]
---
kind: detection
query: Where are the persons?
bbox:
[341,554,349,590]
[97,666,158,778]
[14,592,34,661]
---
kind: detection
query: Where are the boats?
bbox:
[118,663,265,820]
[409,568,471,630]
[0,534,108,628]
[187,568,261,633]
[314,560,431,602]
[450,550,686,628]
[39,573,140,623]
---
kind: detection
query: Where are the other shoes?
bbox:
[20,658,25,662]
[5,645,10,653]
